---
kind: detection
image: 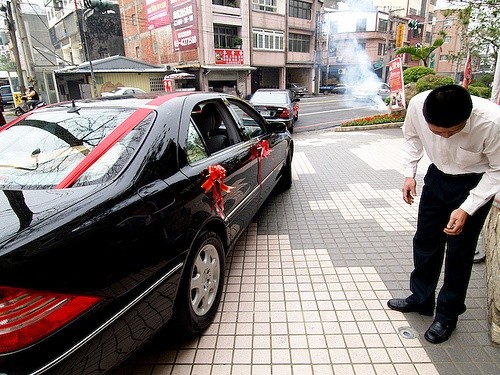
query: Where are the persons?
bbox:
[200,104,223,134]
[24,87,40,109]
[386,85,500,344]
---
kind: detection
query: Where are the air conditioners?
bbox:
[338,69,345,74]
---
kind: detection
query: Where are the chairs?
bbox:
[192,113,228,153]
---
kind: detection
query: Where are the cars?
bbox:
[319,83,348,95]
[354,83,391,103]
[247,88,301,134]
[100,87,146,98]
[0,85,14,105]
[0,90,295,375]
[287,82,309,97]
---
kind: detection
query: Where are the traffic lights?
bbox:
[88,0,101,8]
[102,1,114,10]
[407,19,416,28]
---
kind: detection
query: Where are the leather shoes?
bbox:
[387,298,434,316]
[424,320,456,343]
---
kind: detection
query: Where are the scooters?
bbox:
[14,95,44,116]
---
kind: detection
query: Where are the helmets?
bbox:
[28,85,34,89]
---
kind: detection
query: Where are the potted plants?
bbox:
[232,36,241,49]
